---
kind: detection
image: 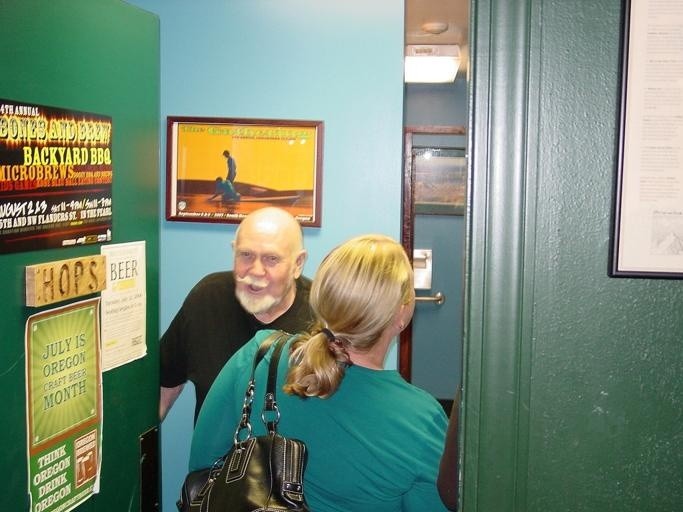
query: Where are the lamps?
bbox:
[404,43,462,85]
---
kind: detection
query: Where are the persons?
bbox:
[205,151,241,206]
[188,236,448,512]
[158,206,314,426]
[437,380,464,512]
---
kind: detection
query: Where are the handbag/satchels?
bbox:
[175,434,309,512]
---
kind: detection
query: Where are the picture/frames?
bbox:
[605,0,683,280]
[411,147,467,216]
[165,116,324,227]
